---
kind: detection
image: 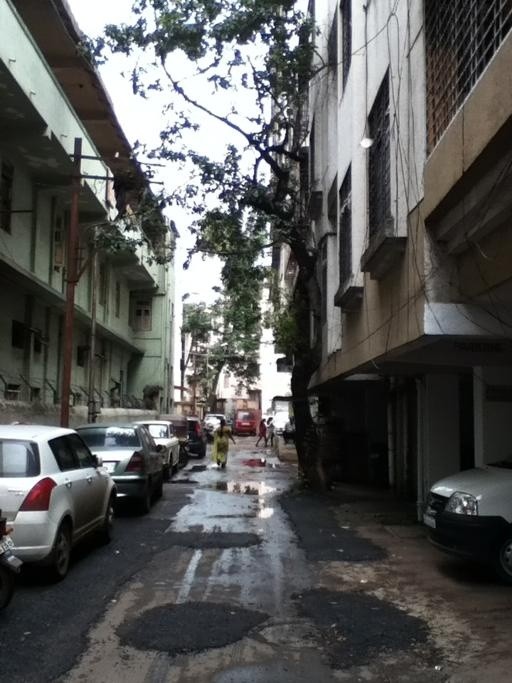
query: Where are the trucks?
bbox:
[232,399,259,436]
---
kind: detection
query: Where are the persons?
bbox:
[213,419,237,468]
[256,417,275,447]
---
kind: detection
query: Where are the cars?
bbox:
[272,412,289,435]
[422,453,512,587]
